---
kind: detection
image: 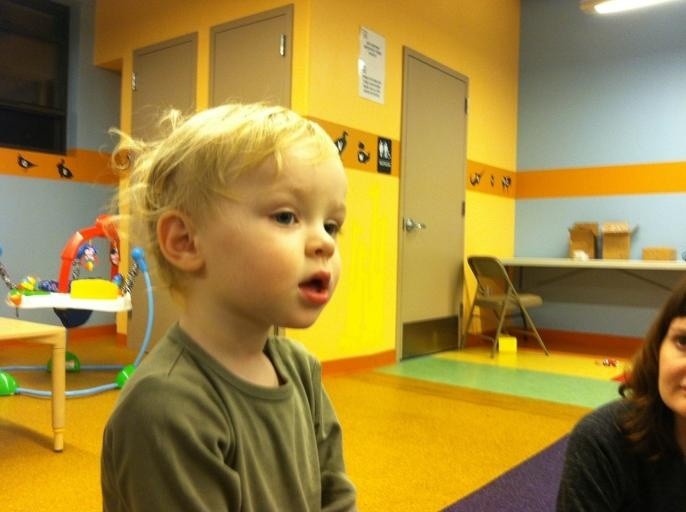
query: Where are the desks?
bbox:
[498,258,686,341]
[0,311,71,453]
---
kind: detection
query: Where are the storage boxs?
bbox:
[566,217,600,260]
[640,245,677,261]
[599,220,639,260]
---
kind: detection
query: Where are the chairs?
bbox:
[458,250,553,361]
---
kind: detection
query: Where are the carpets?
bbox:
[374,341,636,411]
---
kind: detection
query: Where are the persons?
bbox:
[556,287,686,512]
[102,101,356,511]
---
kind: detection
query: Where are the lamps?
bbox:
[580,0,671,16]
